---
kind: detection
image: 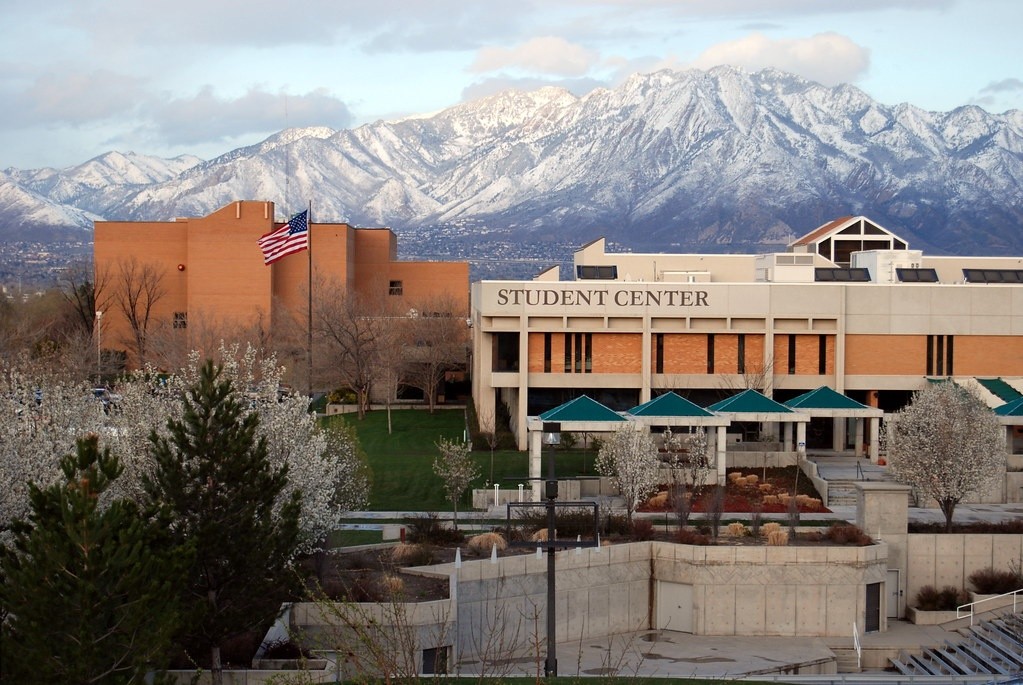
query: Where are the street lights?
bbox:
[96,310,103,385]
[538,422,562,675]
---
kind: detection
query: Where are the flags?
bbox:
[257,209,311,266]
